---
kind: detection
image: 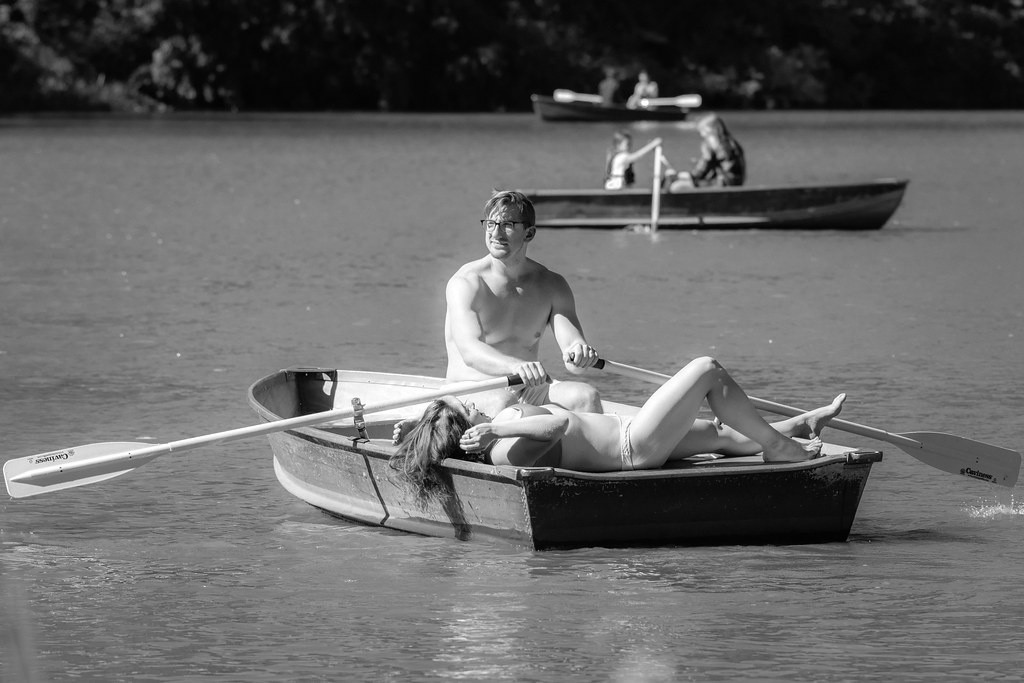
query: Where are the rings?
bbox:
[468,432,471,439]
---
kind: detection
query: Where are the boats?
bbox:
[493,175,911,233]
[246,366,885,554]
[531,93,688,123]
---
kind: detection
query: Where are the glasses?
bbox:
[481,220,528,232]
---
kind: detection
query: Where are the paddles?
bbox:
[553,88,603,104]
[634,94,702,108]
[2,373,524,501]
[572,354,1022,491]
[649,144,663,233]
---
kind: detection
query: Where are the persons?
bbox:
[602,113,746,190]
[597,66,661,112]
[386,356,847,520]
[444,190,603,421]
